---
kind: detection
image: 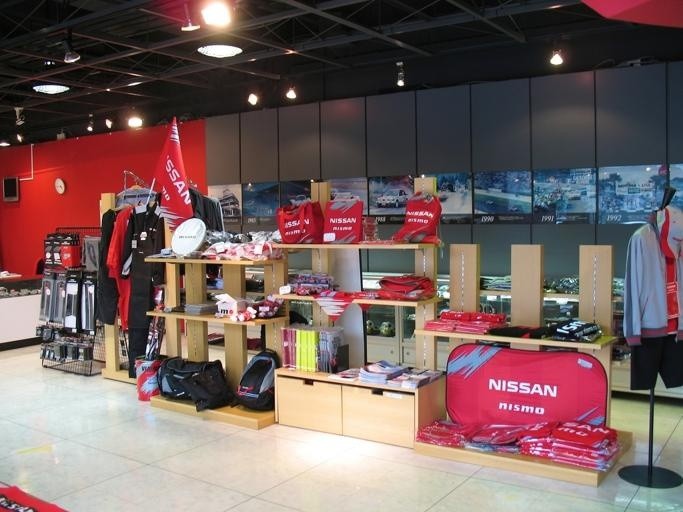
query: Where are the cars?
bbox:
[374,186,410,210]
[560,184,597,202]
[287,193,311,209]
[333,191,354,202]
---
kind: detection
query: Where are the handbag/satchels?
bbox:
[276,200,323,243]
[324,199,362,243]
[157,354,231,412]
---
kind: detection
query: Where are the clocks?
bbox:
[54,177,65,194]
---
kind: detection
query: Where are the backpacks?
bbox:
[234,348,280,410]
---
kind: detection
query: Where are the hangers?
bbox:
[112,197,132,212]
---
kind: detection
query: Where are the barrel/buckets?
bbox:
[136,355,168,401]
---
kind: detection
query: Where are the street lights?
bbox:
[646,166,658,197]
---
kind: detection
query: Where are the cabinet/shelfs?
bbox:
[0,275,44,351]
[416,244,633,485]
[144,218,291,430]
[366,290,683,399]
[274,176,447,448]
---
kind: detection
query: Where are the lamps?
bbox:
[61,29,81,64]
[396,62,405,86]
[286,81,297,99]
[181,2,200,31]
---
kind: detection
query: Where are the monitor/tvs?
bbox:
[2,176,19,201]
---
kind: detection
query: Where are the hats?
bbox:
[657,204,683,261]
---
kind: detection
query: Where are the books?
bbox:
[282,327,442,389]
